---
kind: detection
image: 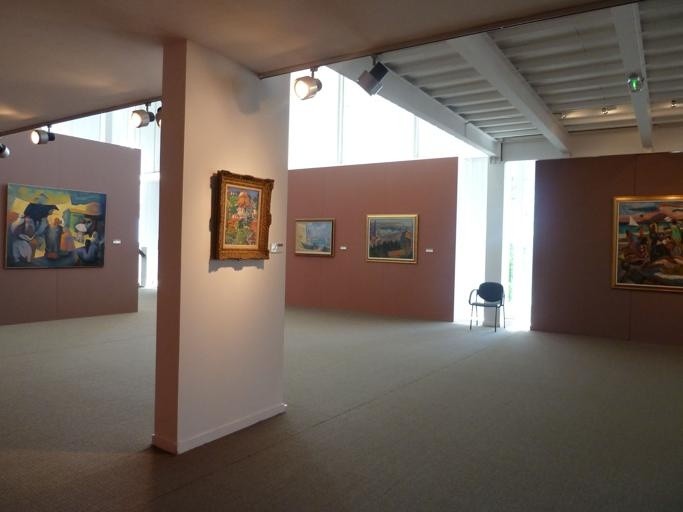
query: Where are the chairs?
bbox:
[467,281,506,332]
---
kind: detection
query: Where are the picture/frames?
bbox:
[610,194,683,293]
[213,170,275,261]
[292,214,420,265]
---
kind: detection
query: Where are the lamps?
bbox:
[130,101,156,127]
[293,57,390,100]
[29,122,56,145]
